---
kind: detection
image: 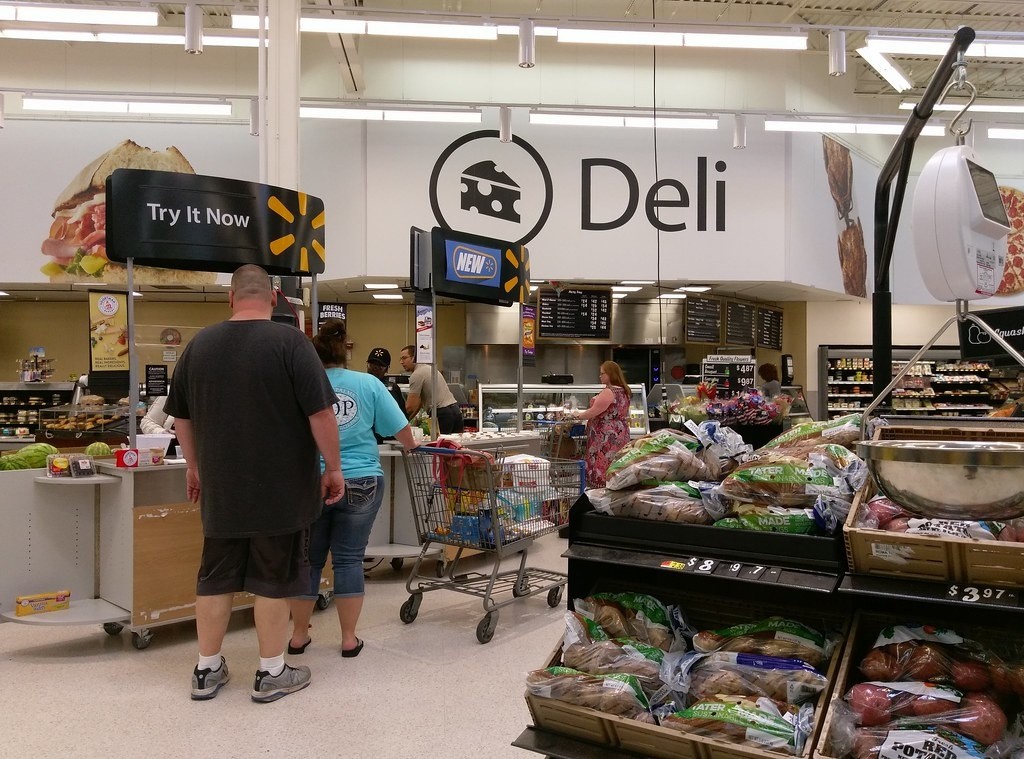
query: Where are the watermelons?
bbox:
[85,441,111,455]
[0,443,60,471]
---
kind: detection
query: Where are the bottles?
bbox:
[459,404,478,418]
[715,366,730,400]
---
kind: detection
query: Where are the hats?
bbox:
[368,348,392,367]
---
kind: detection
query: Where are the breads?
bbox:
[46,395,145,431]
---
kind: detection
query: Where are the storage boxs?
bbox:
[445,424,1024,759]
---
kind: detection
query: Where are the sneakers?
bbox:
[250,664,311,703]
[191,656,230,699]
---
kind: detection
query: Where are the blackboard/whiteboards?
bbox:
[536,285,613,341]
[724,298,756,347]
[757,303,785,352]
[685,292,722,346]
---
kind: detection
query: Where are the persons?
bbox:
[366,348,410,444]
[400,345,464,434]
[163,264,344,703]
[758,363,782,398]
[140,396,179,457]
[572,360,633,487]
[288,319,422,657]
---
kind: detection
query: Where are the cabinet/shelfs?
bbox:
[478,383,651,441]
[827,365,994,421]
[1,472,133,627]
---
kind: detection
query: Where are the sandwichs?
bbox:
[38,137,219,287]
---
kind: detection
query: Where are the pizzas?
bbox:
[995,186,1024,294]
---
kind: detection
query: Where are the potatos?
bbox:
[835,636,1023,759]
[863,497,1024,543]
[595,419,866,535]
[528,605,827,751]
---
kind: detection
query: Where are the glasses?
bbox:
[600,372,606,376]
[367,363,383,371]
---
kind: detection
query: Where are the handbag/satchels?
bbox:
[425,439,494,500]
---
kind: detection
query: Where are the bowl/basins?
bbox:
[127,434,176,458]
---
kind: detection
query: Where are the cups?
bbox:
[410,426,423,442]
[175,444,184,459]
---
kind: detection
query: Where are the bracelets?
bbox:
[576,413,581,419]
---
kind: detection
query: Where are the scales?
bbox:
[852,128,1024,467]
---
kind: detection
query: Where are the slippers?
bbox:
[341,635,364,657]
[288,635,312,655]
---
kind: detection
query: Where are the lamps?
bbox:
[0,0,1024,148]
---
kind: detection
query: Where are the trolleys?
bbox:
[395,444,586,643]
[499,418,574,496]
